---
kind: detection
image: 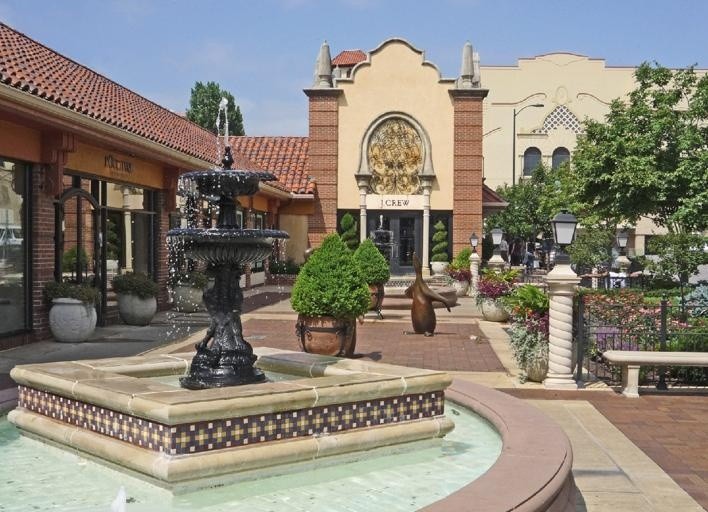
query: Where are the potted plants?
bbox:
[44,280,101,343]
[473,268,520,322]
[427,219,449,277]
[110,271,159,325]
[445,248,472,299]
[289,228,368,358]
[172,270,206,312]
[352,232,389,320]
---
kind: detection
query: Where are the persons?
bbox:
[522,249,536,274]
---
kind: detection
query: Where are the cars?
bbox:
[526,241,555,266]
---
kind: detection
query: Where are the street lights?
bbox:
[615,230,631,266]
[543,209,578,391]
[468,233,481,295]
[512,103,544,190]
[488,228,508,276]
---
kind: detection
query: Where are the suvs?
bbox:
[0,225,23,257]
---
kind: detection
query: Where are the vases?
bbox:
[525,339,577,384]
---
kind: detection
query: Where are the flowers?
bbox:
[494,283,577,385]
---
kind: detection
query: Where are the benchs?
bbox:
[601,348,707,398]
